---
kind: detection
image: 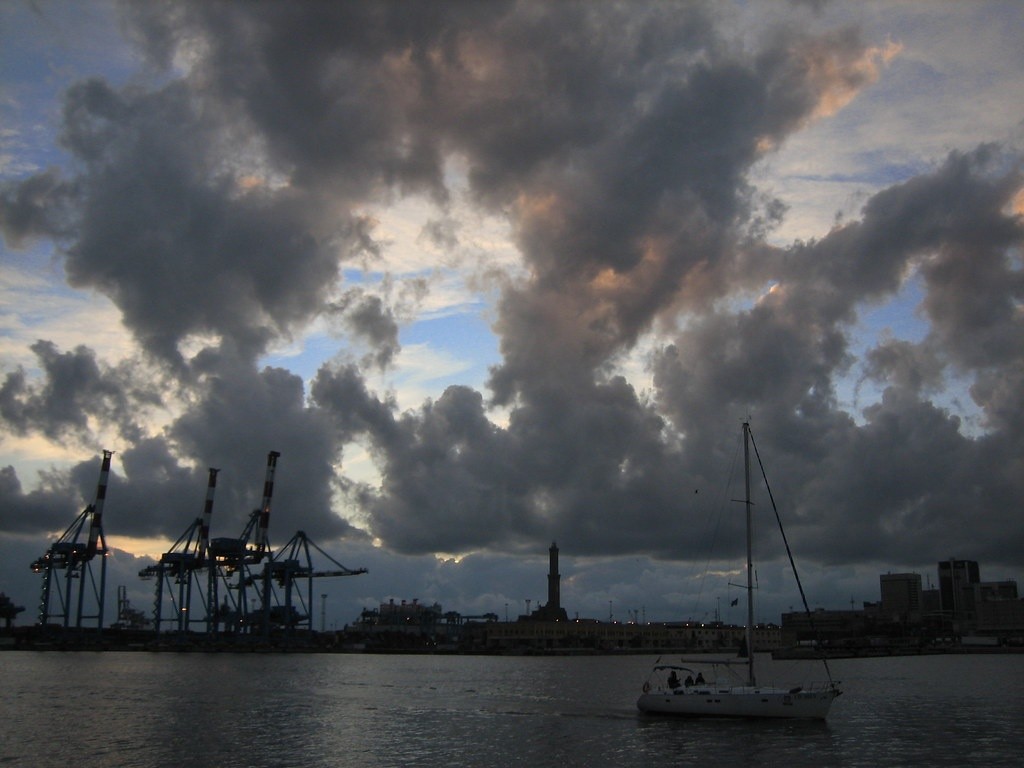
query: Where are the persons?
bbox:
[668,671,705,694]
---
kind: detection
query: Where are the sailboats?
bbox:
[635,413,843,725]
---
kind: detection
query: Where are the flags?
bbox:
[730,598,738,606]
